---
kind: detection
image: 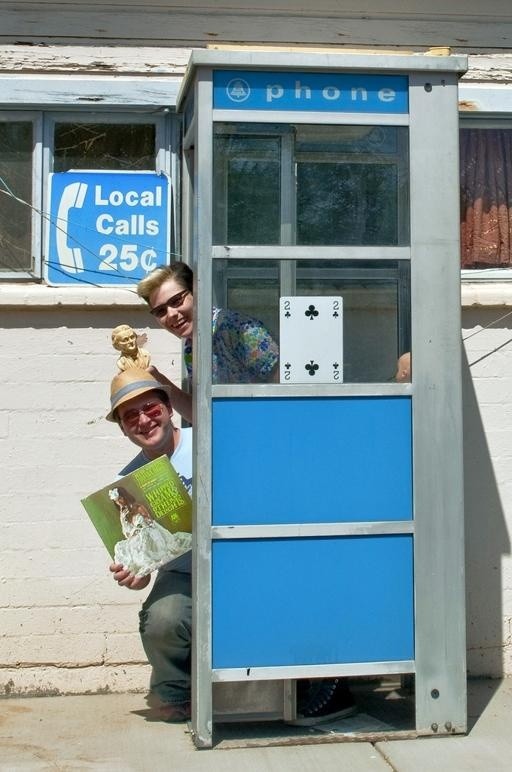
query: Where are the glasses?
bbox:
[149,288,191,318]
[119,399,166,426]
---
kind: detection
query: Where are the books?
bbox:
[81,454,194,579]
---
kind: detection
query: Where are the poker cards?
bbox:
[279,296,343,384]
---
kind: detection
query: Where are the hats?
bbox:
[105,367,172,423]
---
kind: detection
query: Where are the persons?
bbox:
[111,324,149,372]
[133,260,361,729]
[395,351,412,384]
[108,487,193,577]
[102,365,194,723]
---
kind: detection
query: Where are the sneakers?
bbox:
[285,677,358,729]
